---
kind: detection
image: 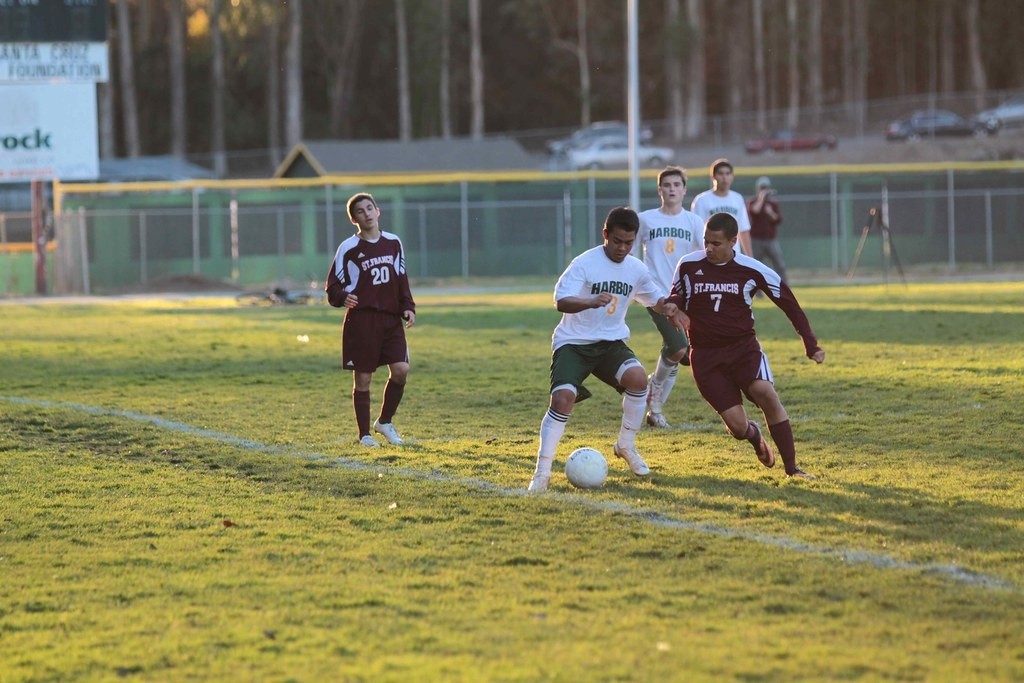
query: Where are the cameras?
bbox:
[763,189,778,202]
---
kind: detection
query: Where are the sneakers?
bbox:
[646,409,671,429]
[528,469,551,491]
[360,435,380,446]
[614,441,649,475]
[787,469,815,480]
[748,419,775,467]
[373,418,403,444]
[647,372,663,414]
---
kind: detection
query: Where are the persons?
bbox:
[528,206,690,492]
[629,166,708,429]
[691,159,753,257]
[745,176,790,297]
[663,212,825,479]
[326,192,416,447]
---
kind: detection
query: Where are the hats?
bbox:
[756,176,771,189]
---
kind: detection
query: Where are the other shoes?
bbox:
[680,343,691,365]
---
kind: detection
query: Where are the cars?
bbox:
[565,135,679,171]
[545,120,658,158]
[0,154,218,244]
[978,96,1024,135]
[744,127,839,158]
[885,108,976,145]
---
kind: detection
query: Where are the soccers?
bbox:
[565,447,610,490]
